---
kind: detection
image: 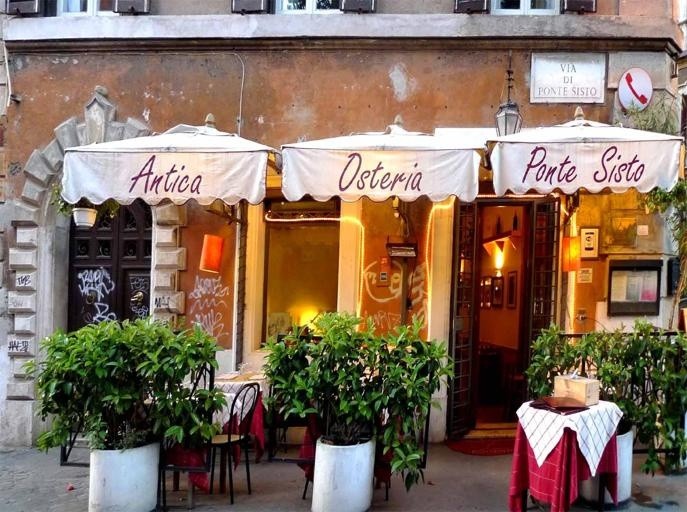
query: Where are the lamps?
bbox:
[562,235,582,272]
[494,102,523,136]
[199,234,224,274]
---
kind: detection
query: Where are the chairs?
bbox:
[208,381,261,506]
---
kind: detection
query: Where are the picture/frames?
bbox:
[506,269,518,310]
[491,276,505,307]
[580,225,602,261]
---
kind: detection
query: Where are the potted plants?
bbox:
[49,179,121,229]
[261,311,455,511]
[524,318,687,510]
[22,320,228,512]
